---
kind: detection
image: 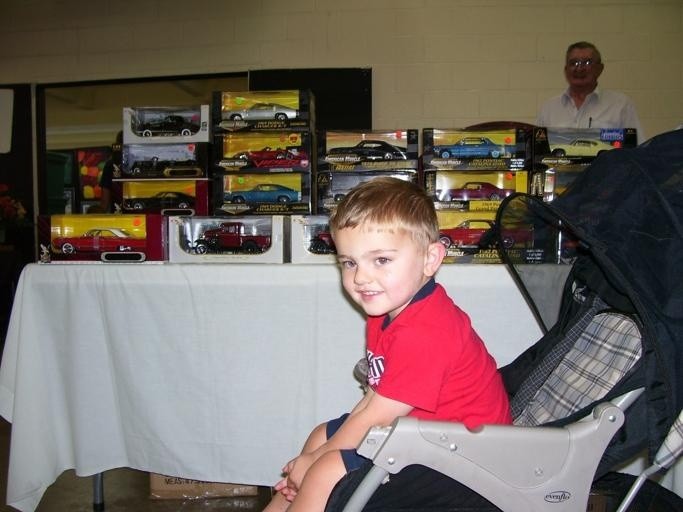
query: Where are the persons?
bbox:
[537,41,642,146]
[258,177,516,512]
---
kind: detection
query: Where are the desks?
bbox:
[26,263,577,507]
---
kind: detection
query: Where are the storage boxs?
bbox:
[147,471,259,502]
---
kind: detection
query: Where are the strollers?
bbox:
[326,130,683,511]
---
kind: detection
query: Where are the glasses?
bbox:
[571,59,593,67]
